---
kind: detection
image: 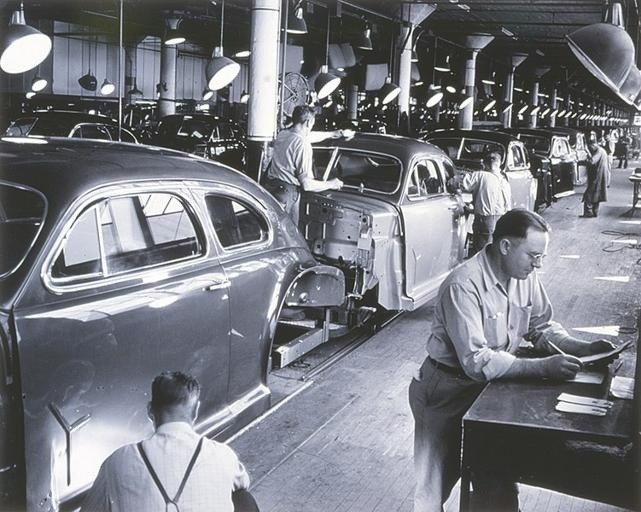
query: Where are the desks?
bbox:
[460,354,641,511]
[627,174,640,217]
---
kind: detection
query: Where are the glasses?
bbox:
[520,246,547,260]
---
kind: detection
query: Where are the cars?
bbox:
[0,137,346,510]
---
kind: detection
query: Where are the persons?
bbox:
[81,368,260,511]
[617,131,628,168]
[603,134,616,188]
[580,141,608,217]
[409,207,618,512]
[445,154,512,260]
[263,107,343,226]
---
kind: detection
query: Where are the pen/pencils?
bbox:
[547,340,566,354]
[614,360,624,373]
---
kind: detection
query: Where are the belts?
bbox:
[426,355,464,377]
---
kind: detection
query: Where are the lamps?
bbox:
[314,6,342,100]
[355,12,373,52]
[425,38,443,108]
[206,0,242,90]
[30,1,47,94]
[455,92,470,107]
[100,44,114,95]
[481,97,496,112]
[410,28,425,62]
[482,70,496,86]
[161,15,186,46]
[129,44,145,99]
[202,87,213,100]
[501,101,512,113]
[515,102,528,115]
[540,104,549,115]
[380,24,401,105]
[231,27,252,60]
[0,1,52,74]
[561,3,636,89]
[528,105,539,115]
[281,0,307,34]
[432,54,451,72]
[77,23,97,92]
[549,108,558,117]
